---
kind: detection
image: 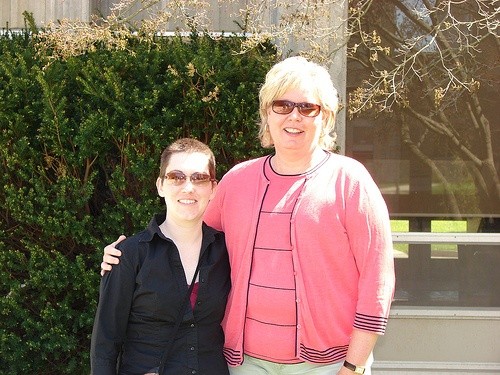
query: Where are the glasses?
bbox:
[159,171,215,186]
[265,100,323,118]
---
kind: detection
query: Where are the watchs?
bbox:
[343,360,365,375]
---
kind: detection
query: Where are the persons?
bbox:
[82,137,237,375]
[100,55,396,375]
